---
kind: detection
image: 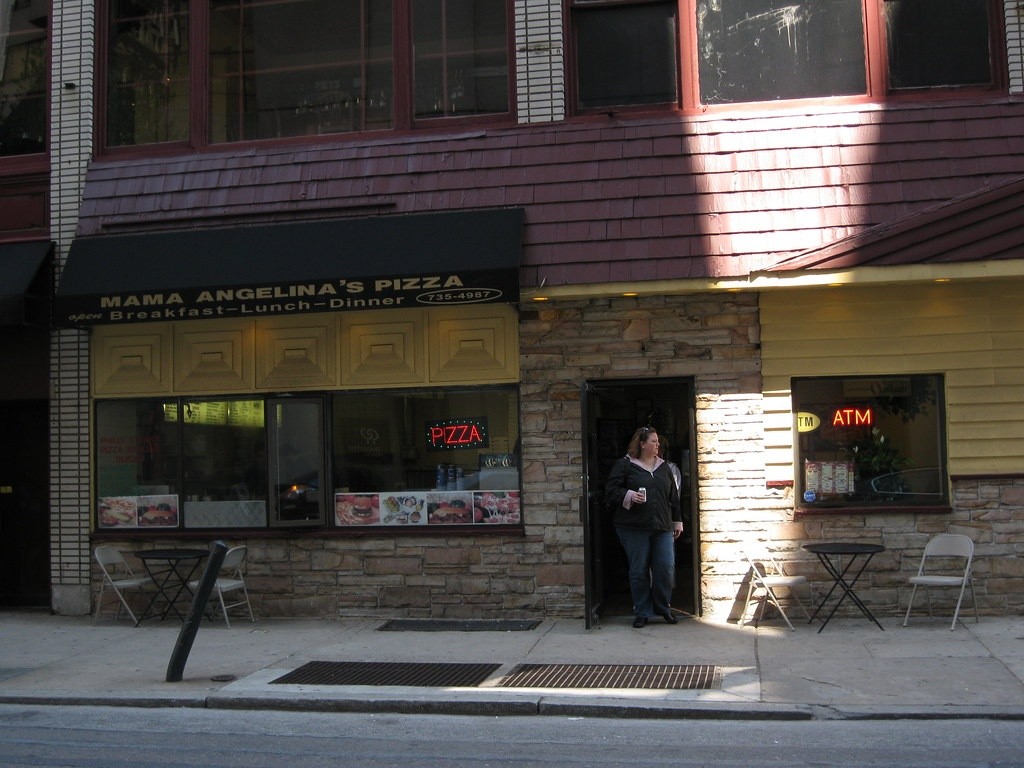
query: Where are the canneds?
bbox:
[638,487,646,502]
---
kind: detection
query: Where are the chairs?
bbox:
[181,546,255,630]
[903,533,980,631]
[93,546,162,627]
[739,540,811,631]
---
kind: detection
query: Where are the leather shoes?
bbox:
[653,608,680,624]
[633,616,648,628]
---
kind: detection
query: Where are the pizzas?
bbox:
[478,493,521,524]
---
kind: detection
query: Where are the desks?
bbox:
[802,542,886,634]
[134,549,213,628]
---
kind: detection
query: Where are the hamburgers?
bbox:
[352,496,373,517]
[139,510,176,525]
[427,506,474,523]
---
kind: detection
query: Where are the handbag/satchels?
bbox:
[603,457,628,512]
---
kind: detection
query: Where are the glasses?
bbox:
[641,427,648,436]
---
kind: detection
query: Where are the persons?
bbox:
[606,425,685,629]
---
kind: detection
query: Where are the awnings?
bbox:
[51,207,528,328]
